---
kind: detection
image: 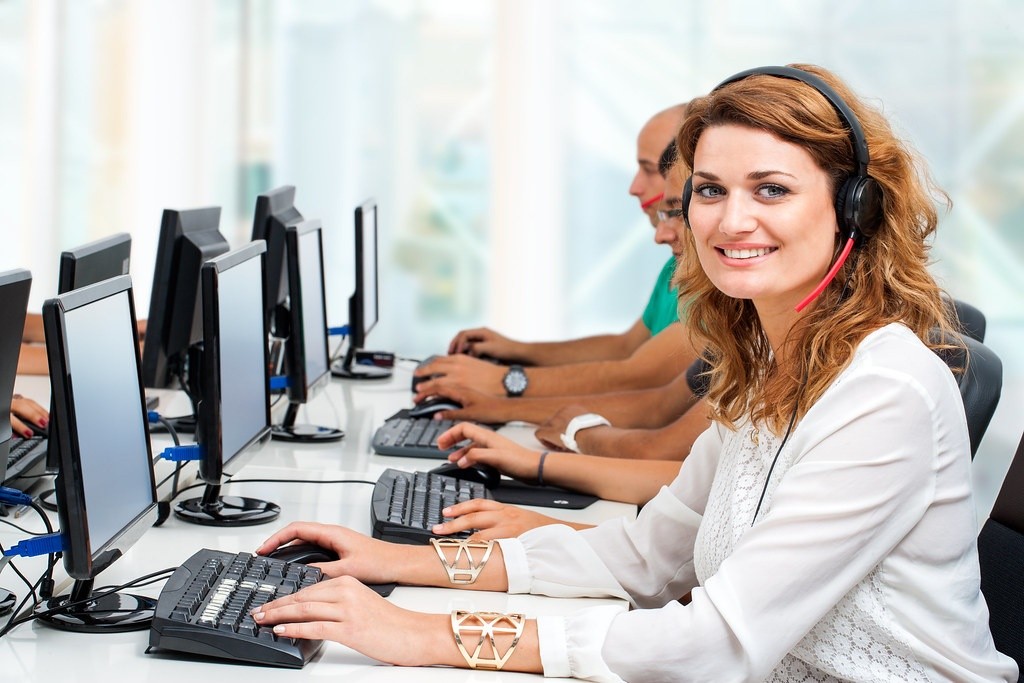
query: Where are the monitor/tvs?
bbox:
[0,183,393,634]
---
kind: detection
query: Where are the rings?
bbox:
[13,394,24,401]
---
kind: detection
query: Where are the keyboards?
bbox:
[412,354,450,394]
[149,548,334,670]
[2,435,49,493]
[371,469,494,545]
[372,417,493,461]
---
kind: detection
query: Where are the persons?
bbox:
[410,101,757,507]
[14,308,148,377]
[9,393,50,439]
[247,63,1019,683]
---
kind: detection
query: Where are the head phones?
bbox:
[682,66,886,246]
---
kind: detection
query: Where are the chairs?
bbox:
[923,293,1003,458]
[975,437,1024,683]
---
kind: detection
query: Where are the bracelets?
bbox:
[451,609,526,670]
[429,535,494,584]
[537,451,550,483]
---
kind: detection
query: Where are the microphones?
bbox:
[795,227,858,313]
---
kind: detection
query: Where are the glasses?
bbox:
[656,208,683,226]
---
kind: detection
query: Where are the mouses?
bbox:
[11,414,49,439]
[265,542,340,564]
[408,397,463,421]
[428,461,501,490]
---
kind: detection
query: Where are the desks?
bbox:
[1,377,640,683]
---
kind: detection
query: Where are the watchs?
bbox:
[560,412,612,454]
[501,365,528,397]
